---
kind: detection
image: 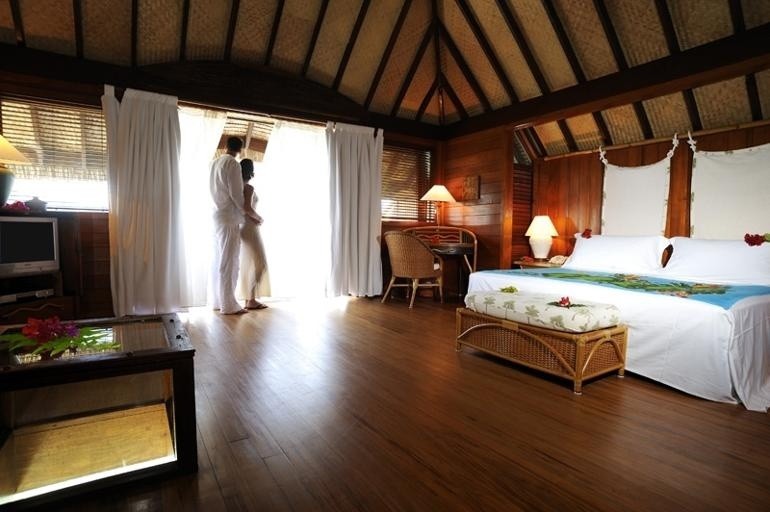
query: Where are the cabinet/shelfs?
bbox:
[0,271,62,297]
[0,297,73,326]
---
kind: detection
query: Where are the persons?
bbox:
[208,136,248,315]
[237,158,271,311]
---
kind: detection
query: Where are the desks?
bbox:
[0,313,197,512]
[400,226,478,304]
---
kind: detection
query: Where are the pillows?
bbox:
[654,237,770,287]
[561,233,670,277]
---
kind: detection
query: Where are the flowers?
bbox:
[0,315,121,357]
[547,296,586,309]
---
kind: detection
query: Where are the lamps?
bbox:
[525,215,559,258]
[420,184,456,226]
[0,135,32,207]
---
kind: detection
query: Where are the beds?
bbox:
[468,247,770,412]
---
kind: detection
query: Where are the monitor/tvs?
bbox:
[0,216,59,277]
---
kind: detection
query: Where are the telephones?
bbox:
[548,255,567,264]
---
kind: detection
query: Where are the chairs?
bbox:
[381,231,444,308]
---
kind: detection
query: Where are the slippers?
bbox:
[223,308,248,315]
[244,303,268,310]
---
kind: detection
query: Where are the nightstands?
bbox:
[514,259,564,269]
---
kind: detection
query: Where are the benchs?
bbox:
[456,290,628,396]
